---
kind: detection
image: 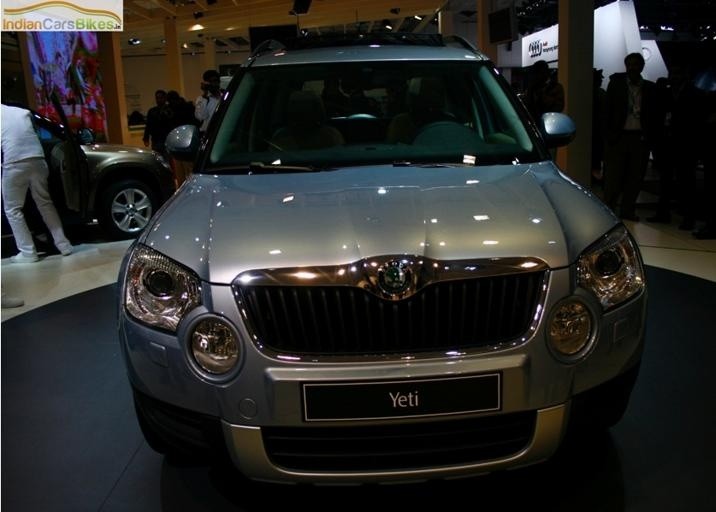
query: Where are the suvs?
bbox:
[113,31,655,488]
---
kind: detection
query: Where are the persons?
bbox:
[1,279,25,308]
[526,61,566,113]
[646,56,706,230]
[142,89,176,177]
[194,70,225,132]
[166,90,204,186]
[694,93,716,239]
[604,52,657,224]
[1,102,75,263]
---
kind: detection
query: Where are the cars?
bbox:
[0,109,177,246]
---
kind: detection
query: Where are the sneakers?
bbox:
[2,293,25,310]
[11,251,40,264]
[60,243,74,255]
